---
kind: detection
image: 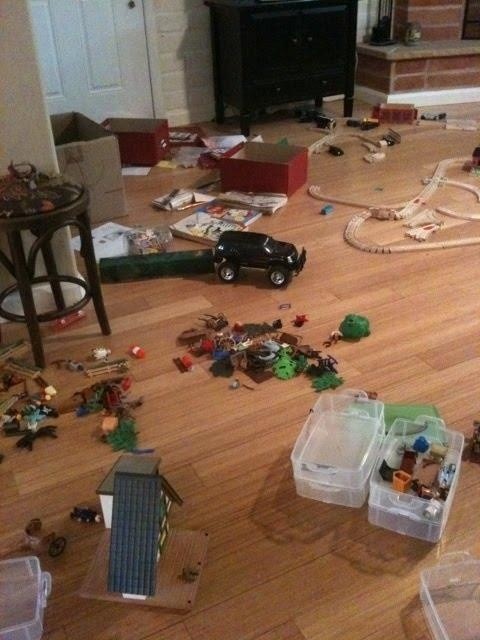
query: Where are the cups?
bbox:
[403,20,422,45]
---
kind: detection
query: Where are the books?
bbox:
[168,200,263,247]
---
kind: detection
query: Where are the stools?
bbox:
[1,170,112,367]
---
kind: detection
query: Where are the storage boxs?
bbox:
[373,103,418,124]
[366,414,465,544]
[219,141,308,195]
[49,110,128,224]
[419,550,480,639]
[0,556,54,639]
[100,118,170,166]
[291,387,386,512]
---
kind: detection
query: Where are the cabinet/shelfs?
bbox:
[209,0,356,132]
